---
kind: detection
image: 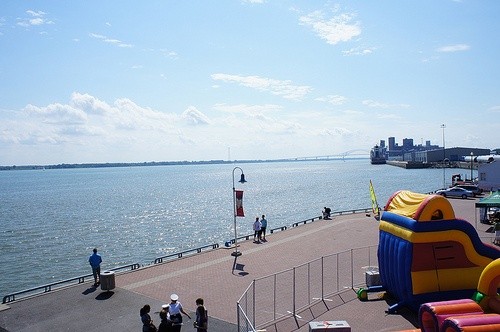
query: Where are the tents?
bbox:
[474,190,500,229]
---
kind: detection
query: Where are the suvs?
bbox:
[449,180,483,196]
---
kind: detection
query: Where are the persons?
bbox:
[491,219,500,245]
[88,248,102,286]
[158,294,192,332]
[252,214,268,244]
[139,304,157,332]
[194,298,208,332]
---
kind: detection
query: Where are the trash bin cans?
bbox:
[100,270,115,290]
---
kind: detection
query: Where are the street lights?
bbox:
[231,166,247,257]
[470,151,474,180]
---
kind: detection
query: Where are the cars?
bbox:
[435,186,475,200]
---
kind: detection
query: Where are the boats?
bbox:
[369,144,387,165]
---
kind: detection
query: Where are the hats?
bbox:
[162,305,169,309]
[171,294,178,303]
